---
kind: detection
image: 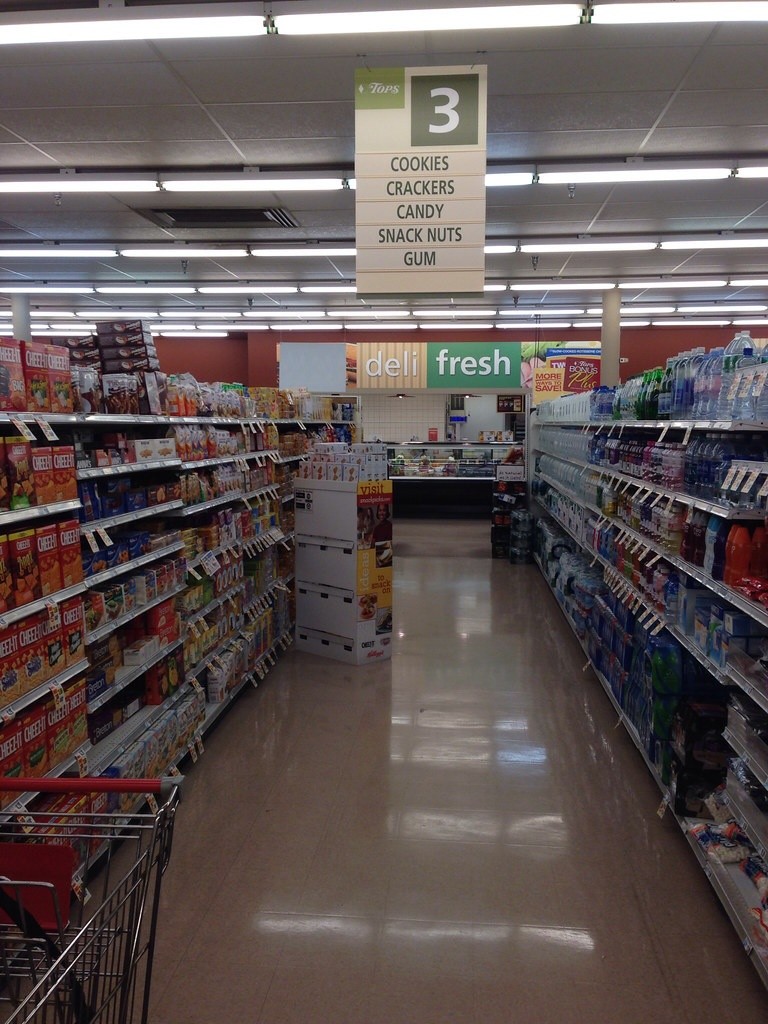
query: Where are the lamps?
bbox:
[0,0,768,331]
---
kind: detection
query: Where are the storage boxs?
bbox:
[293,439,393,666]
[1,336,292,880]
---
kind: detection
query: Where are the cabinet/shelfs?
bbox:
[0,395,363,891]
[490,478,527,560]
[525,394,768,992]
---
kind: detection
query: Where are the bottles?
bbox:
[447,426,453,441]
[511,509,533,531]
[510,546,532,564]
[511,531,533,547]
[265,557,273,584]
[533,330,768,787]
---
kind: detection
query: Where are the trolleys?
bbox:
[1,773,182,1024]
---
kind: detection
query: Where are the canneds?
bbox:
[510,510,532,564]
